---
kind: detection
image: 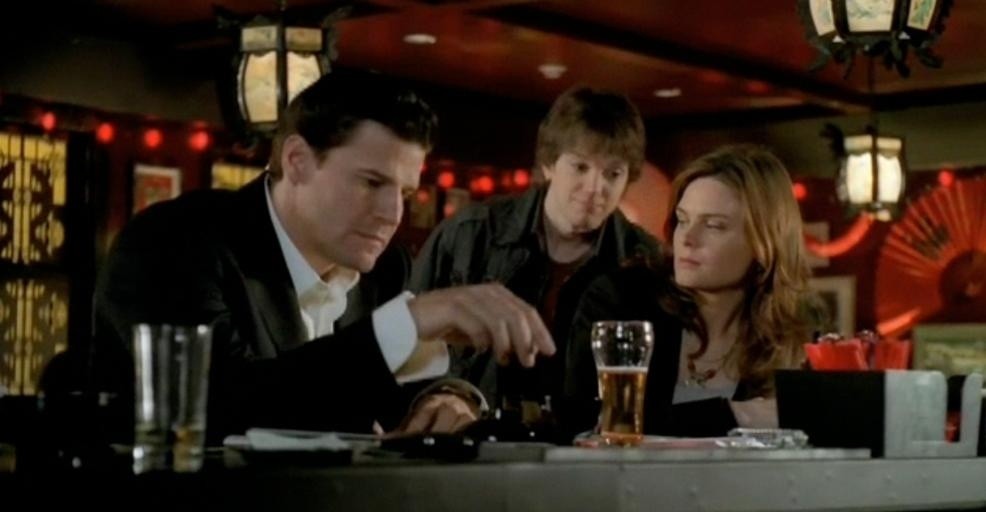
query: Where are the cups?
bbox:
[134,324,213,472]
[594,323,652,444]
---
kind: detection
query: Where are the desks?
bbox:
[185,430,986,512]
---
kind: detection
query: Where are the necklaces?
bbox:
[679,321,748,398]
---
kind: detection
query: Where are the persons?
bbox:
[604,146,833,437]
[88,63,558,436]
[400,86,688,435]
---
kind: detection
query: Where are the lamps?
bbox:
[834,123,906,225]
[225,15,331,137]
[798,0,947,87]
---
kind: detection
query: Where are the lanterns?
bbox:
[834,71,909,217]
[802,1,960,78]
[236,2,335,131]
